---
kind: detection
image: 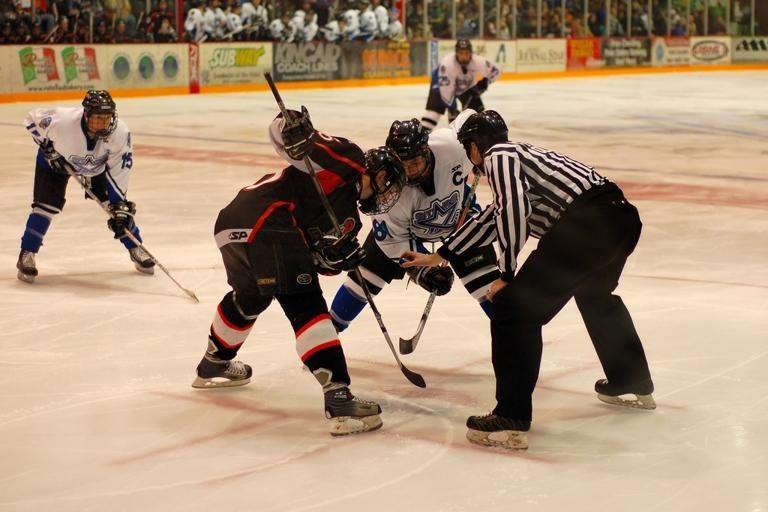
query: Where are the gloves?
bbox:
[448,111,459,123]
[281,117,318,161]
[40,137,66,173]
[470,79,488,95]
[315,234,366,270]
[107,201,136,239]
[406,265,455,296]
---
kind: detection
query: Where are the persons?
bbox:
[192,109,402,420]
[0,1,768,46]
[421,39,500,133]
[402,110,658,433]
[16,87,157,277]
[328,109,513,334]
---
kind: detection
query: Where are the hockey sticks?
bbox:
[262,70,427,389]
[399,173,481,355]
[62,165,201,303]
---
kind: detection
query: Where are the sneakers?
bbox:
[130,245,155,266]
[324,388,381,419]
[17,250,38,275]
[466,413,530,432]
[197,352,252,381]
[595,379,653,396]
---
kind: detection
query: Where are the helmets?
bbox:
[457,110,507,143]
[82,90,118,141]
[456,39,472,47]
[359,118,428,215]
